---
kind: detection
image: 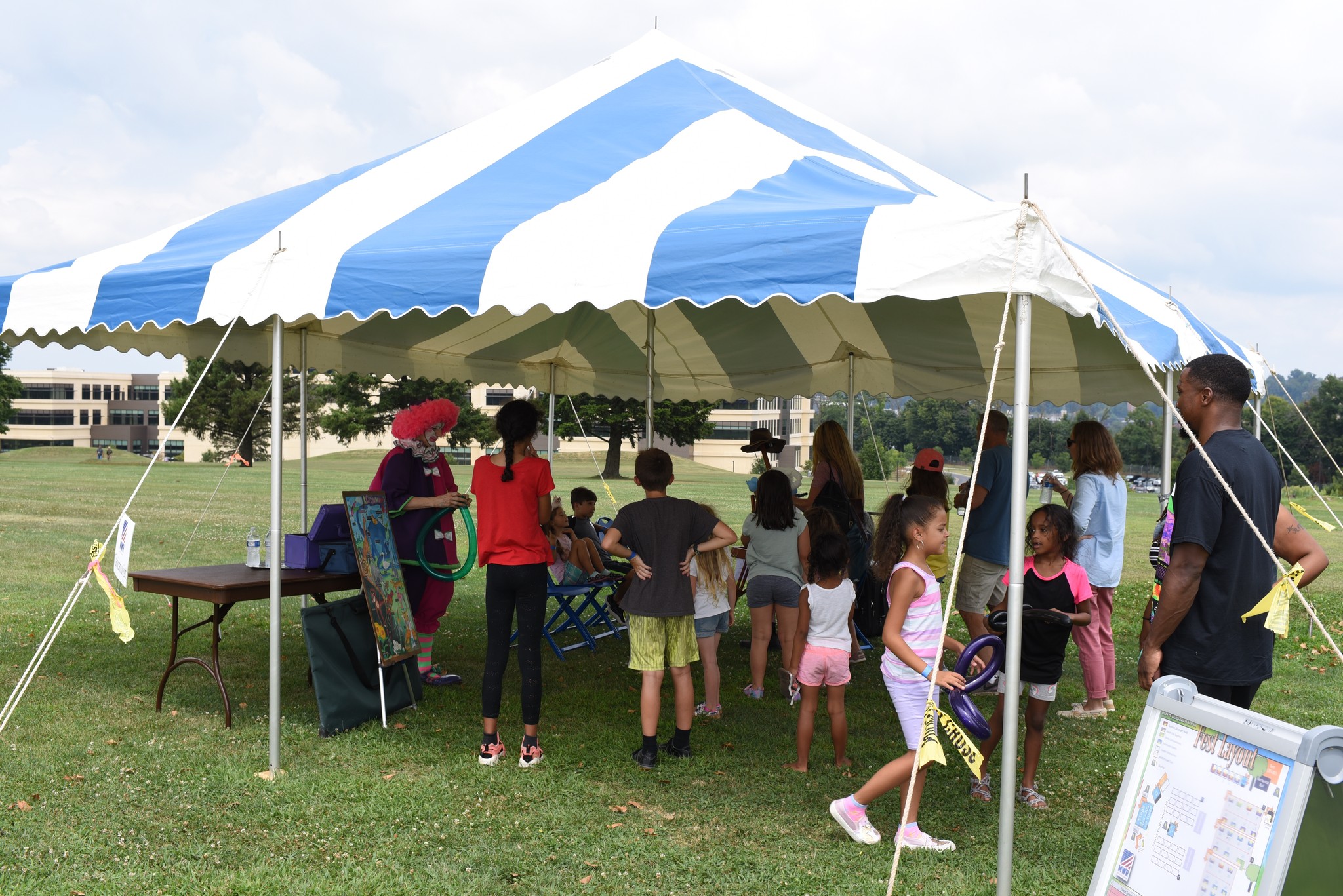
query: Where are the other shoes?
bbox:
[420,666,463,685]
[849,649,866,663]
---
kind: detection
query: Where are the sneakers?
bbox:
[479,731,505,766]
[788,692,800,702]
[893,830,956,852]
[693,702,723,719]
[632,747,656,769]
[743,683,764,700]
[1056,703,1107,721]
[518,735,544,768]
[968,678,999,696]
[829,798,881,845]
[607,572,623,580]
[1071,699,1115,712]
[587,573,610,584]
[659,737,692,759]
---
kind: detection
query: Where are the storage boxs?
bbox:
[284,503,350,570]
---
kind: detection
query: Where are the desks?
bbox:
[128,560,364,731]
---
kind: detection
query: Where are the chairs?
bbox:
[508,516,627,663]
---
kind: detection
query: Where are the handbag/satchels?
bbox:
[843,520,874,586]
[814,474,850,530]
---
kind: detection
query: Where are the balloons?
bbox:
[746,467,803,496]
[987,602,1072,631]
[416,496,477,581]
[942,634,1005,740]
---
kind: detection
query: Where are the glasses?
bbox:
[1066,438,1076,447]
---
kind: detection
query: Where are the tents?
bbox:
[0,16,1343,896]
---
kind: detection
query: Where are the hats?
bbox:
[910,449,944,473]
[741,428,787,455]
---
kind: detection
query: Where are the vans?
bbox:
[1123,474,1142,482]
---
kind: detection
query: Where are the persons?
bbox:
[96,445,104,460]
[953,410,1029,696]
[792,420,866,663]
[970,503,1093,809]
[106,446,113,460]
[470,399,556,768]
[1140,436,1328,653]
[601,447,738,769]
[563,486,635,625]
[741,469,811,701]
[689,504,736,720]
[828,493,985,853]
[367,397,473,685]
[541,494,623,585]
[1137,353,1282,710]
[1040,421,1128,720]
[781,530,856,773]
[900,448,953,584]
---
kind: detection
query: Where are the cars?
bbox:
[1133,476,1162,486]
[1028,469,1066,486]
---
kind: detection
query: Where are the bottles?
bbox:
[247,527,260,566]
[264,527,271,568]
[957,485,968,516]
[1039,468,1055,505]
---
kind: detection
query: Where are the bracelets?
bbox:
[626,550,636,560]
[983,615,989,617]
[921,665,933,678]
[1060,489,1068,494]
[1142,617,1151,620]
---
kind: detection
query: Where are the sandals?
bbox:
[778,668,800,698]
[1016,783,1048,810]
[970,772,991,803]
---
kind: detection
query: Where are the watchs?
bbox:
[694,543,703,555]
